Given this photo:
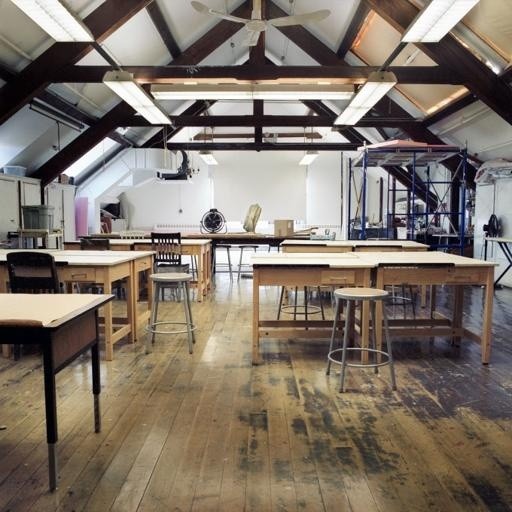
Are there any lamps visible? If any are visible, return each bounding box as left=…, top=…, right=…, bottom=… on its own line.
left=399, top=0, right=480, bottom=45
left=199, top=122, right=220, bottom=167
left=102, top=71, right=172, bottom=126
left=332, top=73, right=397, bottom=128
left=299, top=125, right=317, bottom=164
left=10, top=0, right=95, bottom=42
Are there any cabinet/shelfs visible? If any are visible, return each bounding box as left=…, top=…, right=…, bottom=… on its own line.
left=46, top=184, right=77, bottom=241
left=466, top=174, right=511, bottom=289
left=0, top=173, right=43, bottom=244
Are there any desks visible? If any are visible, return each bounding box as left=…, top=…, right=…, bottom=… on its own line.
left=0, top=290, right=120, bottom=493
left=238, top=203, right=511, bottom=366
left=1, top=248, right=157, bottom=359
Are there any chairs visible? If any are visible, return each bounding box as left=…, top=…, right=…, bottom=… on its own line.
left=5, top=251, right=65, bottom=364
left=62, top=226, right=214, bottom=303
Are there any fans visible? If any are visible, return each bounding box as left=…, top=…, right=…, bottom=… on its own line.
left=200, top=208, right=227, bottom=234
left=191, top=1, right=331, bottom=47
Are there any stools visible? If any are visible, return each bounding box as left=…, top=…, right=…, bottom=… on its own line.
left=214, top=244, right=234, bottom=283
left=148, top=272, right=198, bottom=355
left=325, top=287, right=402, bottom=393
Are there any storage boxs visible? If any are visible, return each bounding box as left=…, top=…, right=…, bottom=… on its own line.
left=3, top=165, right=27, bottom=177
left=22, top=204, right=55, bottom=234
